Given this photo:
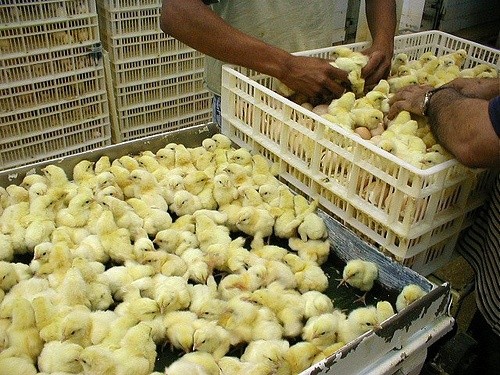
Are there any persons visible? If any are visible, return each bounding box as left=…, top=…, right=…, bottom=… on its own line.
left=386, top=75, right=500, bottom=374
left=156, top=1, right=399, bottom=134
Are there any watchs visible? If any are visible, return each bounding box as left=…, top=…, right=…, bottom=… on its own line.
left=419, top=86, right=449, bottom=118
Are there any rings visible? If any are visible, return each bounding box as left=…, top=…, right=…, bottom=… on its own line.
left=342, top=88, right=348, bottom=95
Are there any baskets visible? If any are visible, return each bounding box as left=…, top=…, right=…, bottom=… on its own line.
left=221, top=30, right=500, bottom=277
left=1, top=0, right=213, bottom=170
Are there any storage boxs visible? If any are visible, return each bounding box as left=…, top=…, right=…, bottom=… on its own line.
left=1, top=0, right=213, bottom=169
left=220, top=30, right=499, bottom=279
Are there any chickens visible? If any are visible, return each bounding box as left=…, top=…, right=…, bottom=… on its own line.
left=235, top=45, right=499, bottom=219
left=0, top=135, right=428, bottom=375
